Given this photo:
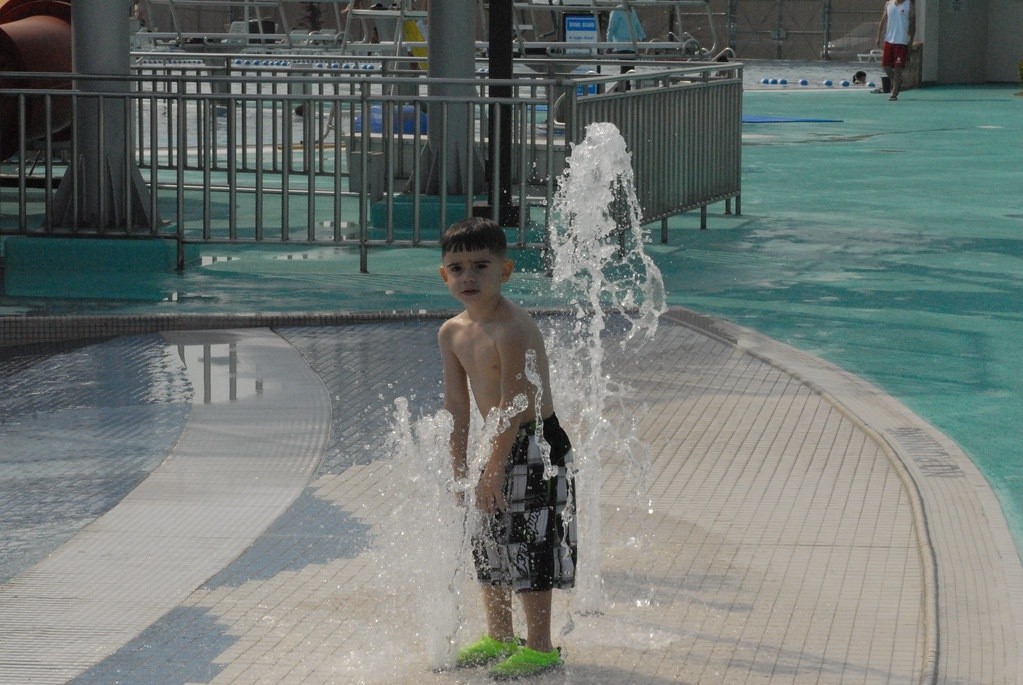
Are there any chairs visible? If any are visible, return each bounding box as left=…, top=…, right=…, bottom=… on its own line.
left=228, top=21, right=247, bottom=43
left=289, top=28, right=337, bottom=47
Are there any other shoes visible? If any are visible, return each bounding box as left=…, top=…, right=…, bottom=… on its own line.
left=888, top=96, right=897, bottom=101
left=486, top=646, right=564, bottom=681
left=456, top=633, right=527, bottom=669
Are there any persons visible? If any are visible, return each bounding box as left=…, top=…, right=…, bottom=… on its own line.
left=605, top=0, right=646, bottom=74
left=437, top=216, right=578, bottom=676
left=853, top=71, right=866, bottom=84
left=876, top=0, right=915, bottom=101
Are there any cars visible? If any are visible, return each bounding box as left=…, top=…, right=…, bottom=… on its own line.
left=176, top=37, right=220, bottom=49
left=820, top=20, right=881, bottom=60
